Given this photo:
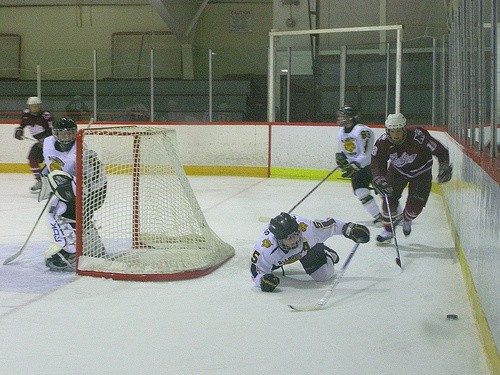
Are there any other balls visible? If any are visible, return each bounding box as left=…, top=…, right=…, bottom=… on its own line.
left=446, top=314, right=458, bottom=320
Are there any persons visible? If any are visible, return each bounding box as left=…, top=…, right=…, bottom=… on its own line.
left=250, top=213, right=370, bottom=292
left=370, top=114, right=453, bottom=242
left=15, top=97, right=106, bottom=271
left=335, top=105, right=404, bottom=228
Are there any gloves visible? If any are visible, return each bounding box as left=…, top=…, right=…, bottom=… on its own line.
left=437, top=161, right=452, bottom=183
left=54, top=180, right=75, bottom=202
left=371, top=176, right=393, bottom=197
left=15, top=126, right=23, bottom=140
left=260, top=273, right=280, bottom=292
left=32, top=132, right=44, bottom=139
left=335, top=151, right=360, bottom=178
left=342, top=221, right=370, bottom=244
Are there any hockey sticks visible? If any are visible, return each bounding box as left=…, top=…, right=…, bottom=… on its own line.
left=3, top=193, right=54, bottom=266
left=21, top=119, right=95, bottom=144
left=384, top=194, right=401, bottom=266
left=256, top=164, right=339, bottom=224
left=287, top=237, right=362, bottom=310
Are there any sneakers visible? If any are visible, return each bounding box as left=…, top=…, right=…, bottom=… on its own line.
left=323, top=245, right=339, bottom=264
left=45, top=248, right=82, bottom=272
left=29, top=176, right=42, bottom=194
left=376, top=219, right=396, bottom=243
left=402, top=216, right=412, bottom=237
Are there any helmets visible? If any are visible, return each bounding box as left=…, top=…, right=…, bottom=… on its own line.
left=268, top=212, right=301, bottom=254
left=26, top=97, right=42, bottom=105
left=52, top=116, right=77, bottom=151
left=385, top=113, right=407, bottom=144
left=335, top=104, right=353, bottom=127
